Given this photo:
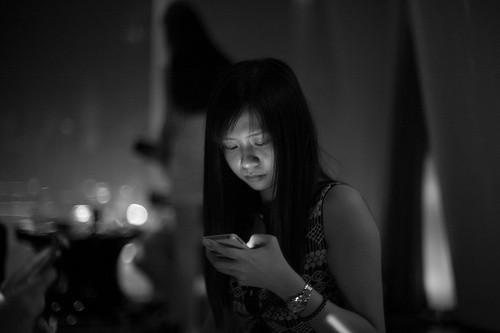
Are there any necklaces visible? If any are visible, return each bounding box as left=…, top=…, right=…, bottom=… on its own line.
left=255, top=207, right=264, bottom=219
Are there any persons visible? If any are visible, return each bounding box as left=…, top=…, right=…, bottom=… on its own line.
left=157, top=0, right=231, bottom=330
left=201, top=56, right=386, bottom=332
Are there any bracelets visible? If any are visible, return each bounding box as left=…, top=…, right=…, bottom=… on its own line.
left=300, top=294, right=328, bottom=322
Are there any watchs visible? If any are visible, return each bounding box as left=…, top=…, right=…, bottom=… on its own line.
left=286, top=283, right=312, bottom=314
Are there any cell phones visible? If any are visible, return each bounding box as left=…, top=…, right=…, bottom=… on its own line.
left=201, top=233, right=250, bottom=258
